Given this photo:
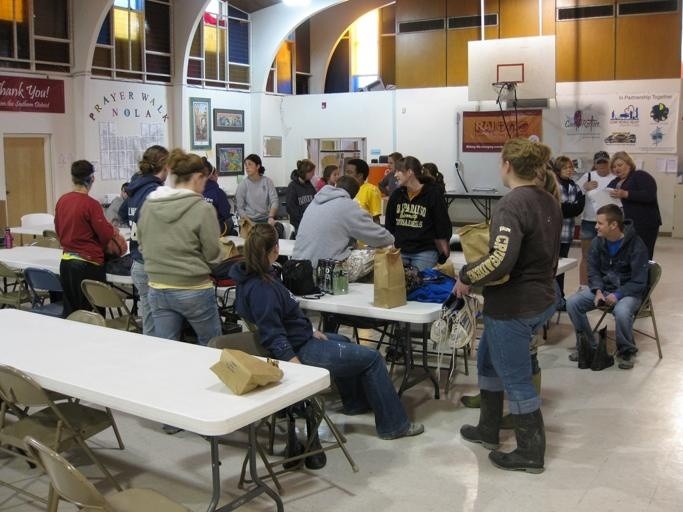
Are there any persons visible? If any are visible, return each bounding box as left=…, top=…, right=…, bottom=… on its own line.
left=104, top=143, right=453, bottom=364
left=229, top=221, right=426, bottom=441
left=137, top=149, right=226, bottom=436
left=575, top=150, right=624, bottom=293
left=451, top=137, right=563, bottom=474
left=550, top=157, right=584, bottom=313
left=563, top=202, right=648, bottom=370
left=455, top=142, right=560, bottom=429
left=606, top=150, right=662, bottom=262
left=51, top=158, right=119, bottom=321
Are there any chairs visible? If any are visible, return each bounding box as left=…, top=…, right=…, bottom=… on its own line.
left=593, top=260, right=663, bottom=360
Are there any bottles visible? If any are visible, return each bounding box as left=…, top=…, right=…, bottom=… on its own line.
left=316, top=258, right=350, bottom=297
left=4, top=228, right=12, bottom=249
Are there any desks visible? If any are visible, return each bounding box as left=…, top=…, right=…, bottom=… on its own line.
left=443, top=189, right=504, bottom=225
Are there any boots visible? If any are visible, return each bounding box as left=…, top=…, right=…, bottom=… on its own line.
left=589, top=323, right=615, bottom=371
left=460, top=393, right=482, bottom=409
left=576, top=324, right=595, bottom=370
left=502, top=368, right=541, bottom=430
left=303, top=400, right=328, bottom=471
left=280, top=402, right=306, bottom=472
left=489, top=408, right=546, bottom=475
left=461, top=388, right=504, bottom=450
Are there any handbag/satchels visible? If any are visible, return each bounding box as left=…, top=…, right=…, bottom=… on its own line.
left=281, top=258, right=322, bottom=296
left=104, top=231, right=129, bottom=258
left=401, top=263, right=425, bottom=297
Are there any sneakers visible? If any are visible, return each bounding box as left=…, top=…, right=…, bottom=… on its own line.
left=382, top=422, right=425, bottom=440
left=568, top=351, right=580, bottom=362
left=618, top=347, right=634, bottom=369
left=384, top=348, right=404, bottom=365
left=447, top=292, right=488, bottom=349
left=163, top=423, right=183, bottom=434
left=428, top=291, right=459, bottom=344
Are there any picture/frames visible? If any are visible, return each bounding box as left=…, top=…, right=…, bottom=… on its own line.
left=212, top=108, right=245, bottom=132
left=216, top=143, right=245, bottom=176
left=189, top=97, right=212, bottom=151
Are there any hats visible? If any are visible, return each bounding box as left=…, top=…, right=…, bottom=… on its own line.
left=594, top=151, right=610, bottom=162
